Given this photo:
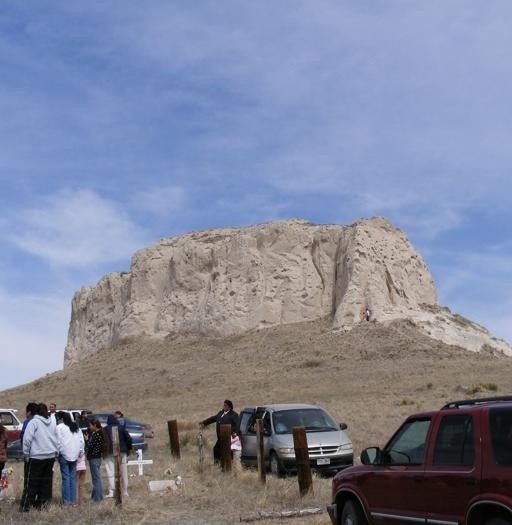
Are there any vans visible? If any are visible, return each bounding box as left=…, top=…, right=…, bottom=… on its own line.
left=234, top=402, right=357, bottom=479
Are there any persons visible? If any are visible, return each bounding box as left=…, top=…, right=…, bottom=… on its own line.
left=231, top=430, right=242, bottom=467
left=253, top=423, right=257, bottom=433
left=199, top=400, right=240, bottom=464
left=0, top=402, right=133, bottom=512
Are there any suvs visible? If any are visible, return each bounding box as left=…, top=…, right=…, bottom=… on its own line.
left=322, top=391, right=512, bottom=525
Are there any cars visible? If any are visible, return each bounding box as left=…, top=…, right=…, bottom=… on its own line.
left=0, top=408, right=155, bottom=461
